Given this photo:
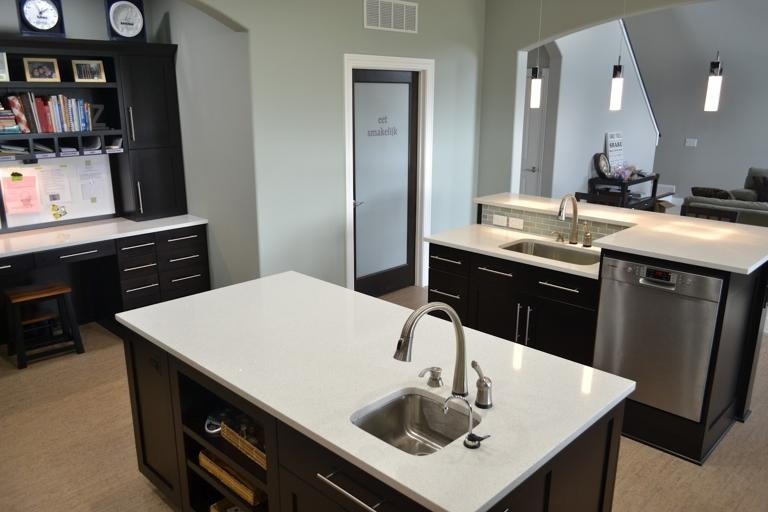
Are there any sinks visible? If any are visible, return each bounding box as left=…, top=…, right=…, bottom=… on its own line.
left=498, top=238, right=602, bottom=266
left=349, top=387, right=481, bottom=455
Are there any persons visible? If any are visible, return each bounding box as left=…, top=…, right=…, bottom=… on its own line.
left=31, top=65, right=56, bottom=78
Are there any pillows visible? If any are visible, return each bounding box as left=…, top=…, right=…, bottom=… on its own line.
left=691, top=187, right=735, bottom=200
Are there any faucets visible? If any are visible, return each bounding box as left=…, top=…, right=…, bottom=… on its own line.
left=555, top=194, right=579, bottom=244
left=393, top=300, right=469, bottom=398
left=442, top=396, right=492, bottom=449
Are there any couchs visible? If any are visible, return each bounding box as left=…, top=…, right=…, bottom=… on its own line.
left=679, top=162, right=767, bottom=231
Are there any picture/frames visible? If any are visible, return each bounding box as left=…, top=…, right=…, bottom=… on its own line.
left=73, top=59, right=109, bottom=85
left=22, top=55, right=61, bottom=85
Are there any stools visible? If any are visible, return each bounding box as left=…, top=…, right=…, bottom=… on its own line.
left=0, top=281, right=85, bottom=368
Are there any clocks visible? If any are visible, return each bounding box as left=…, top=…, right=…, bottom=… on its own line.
left=15, top=0, right=67, bottom=39
left=594, top=153, right=611, bottom=176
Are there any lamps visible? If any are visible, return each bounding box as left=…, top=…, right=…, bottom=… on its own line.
left=701, top=0, right=727, bottom=114
left=606, top=0, right=627, bottom=112
left=528, top=0, right=544, bottom=110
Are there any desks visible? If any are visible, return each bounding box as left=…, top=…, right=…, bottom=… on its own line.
left=0, top=220, right=211, bottom=362
left=588, top=171, right=660, bottom=211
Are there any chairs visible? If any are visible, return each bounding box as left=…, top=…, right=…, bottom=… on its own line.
left=680, top=202, right=740, bottom=223
left=575, top=191, right=621, bottom=208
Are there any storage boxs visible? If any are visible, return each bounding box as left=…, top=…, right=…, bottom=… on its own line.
left=631, top=193, right=675, bottom=215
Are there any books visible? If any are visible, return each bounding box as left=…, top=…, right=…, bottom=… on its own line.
left=0, top=89, right=123, bottom=157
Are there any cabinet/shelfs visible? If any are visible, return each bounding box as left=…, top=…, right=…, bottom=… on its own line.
left=166, top=355, right=277, bottom=512
left=463, top=251, right=603, bottom=367
left=122, top=329, right=181, bottom=512
left=120, top=43, right=188, bottom=224
left=0, top=40, right=125, bottom=169
left=427, top=244, right=468, bottom=326
left=277, top=422, right=435, bottom=512
